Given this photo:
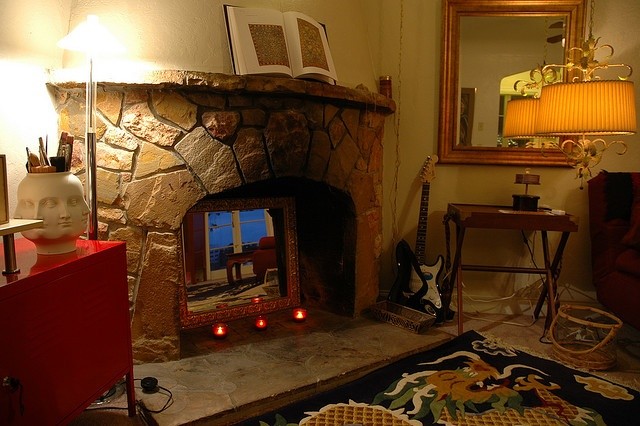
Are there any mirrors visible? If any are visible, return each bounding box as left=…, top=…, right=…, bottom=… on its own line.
left=437, top=1, right=591, bottom=168
left=179, top=196, right=301, bottom=330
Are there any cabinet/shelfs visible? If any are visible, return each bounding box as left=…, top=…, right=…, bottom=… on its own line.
left=0, top=233, right=136, bottom=426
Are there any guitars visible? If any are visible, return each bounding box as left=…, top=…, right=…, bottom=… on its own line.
left=388, top=154, right=445, bottom=316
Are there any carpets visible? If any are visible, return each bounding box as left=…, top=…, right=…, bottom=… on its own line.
left=183, top=329, right=640, bottom=425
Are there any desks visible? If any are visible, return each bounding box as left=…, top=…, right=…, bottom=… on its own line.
left=445, top=198, right=579, bottom=336
left=226, top=250, right=254, bottom=286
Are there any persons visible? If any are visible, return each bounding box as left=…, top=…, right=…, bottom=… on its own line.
left=13, top=173, right=91, bottom=255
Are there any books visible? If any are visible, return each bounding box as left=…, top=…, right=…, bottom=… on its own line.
left=223, top=5, right=338, bottom=84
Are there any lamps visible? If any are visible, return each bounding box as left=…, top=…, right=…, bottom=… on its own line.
left=56, top=14, right=125, bottom=242
left=503, top=16, right=556, bottom=142
left=533, top=1, right=638, bottom=191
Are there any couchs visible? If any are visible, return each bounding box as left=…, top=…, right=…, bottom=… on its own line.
left=253, top=235, right=278, bottom=275
left=588, top=171, right=640, bottom=329
